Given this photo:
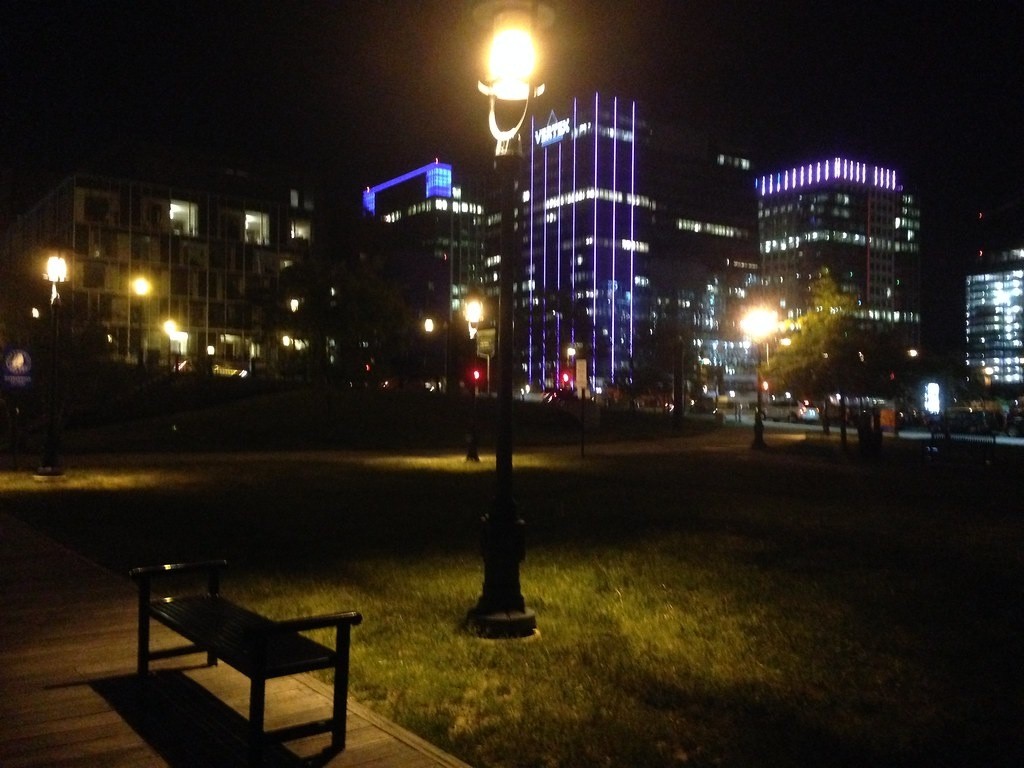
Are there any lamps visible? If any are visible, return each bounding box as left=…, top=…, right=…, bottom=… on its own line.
left=46, top=256, right=66, bottom=282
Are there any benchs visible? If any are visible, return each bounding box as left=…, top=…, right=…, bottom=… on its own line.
left=130, top=559, right=363, bottom=768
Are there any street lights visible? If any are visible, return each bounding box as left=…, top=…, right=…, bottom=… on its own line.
left=37, top=256, right=72, bottom=478
left=131, top=277, right=150, bottom=363
left=473, top=19, right=543, bottom=638
left=743, top=305, right=779, bottom=450
